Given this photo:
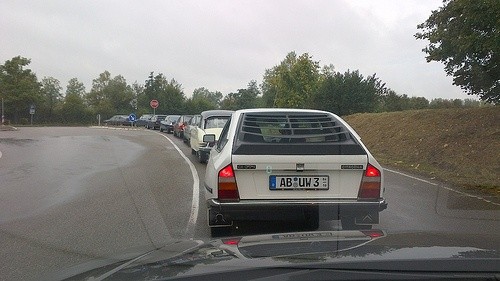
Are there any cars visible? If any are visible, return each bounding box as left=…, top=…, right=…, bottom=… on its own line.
left=204, top=108, right=387, bottom=238
left=182, top=114, right=202, bottom=145
left=172, top=114, right=194, bottom=139
left=160, top=115, right=181, bottom=134
left=144, top=115, right=166, bottom=130
left=190, top=109, right=236, bottom=163
left=107, top=115, right=132, bottom=126
left=134, top=115, right=155, bottom=128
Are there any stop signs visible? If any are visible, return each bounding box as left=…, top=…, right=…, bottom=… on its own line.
left=150, top=100, right=159, bottom=108
